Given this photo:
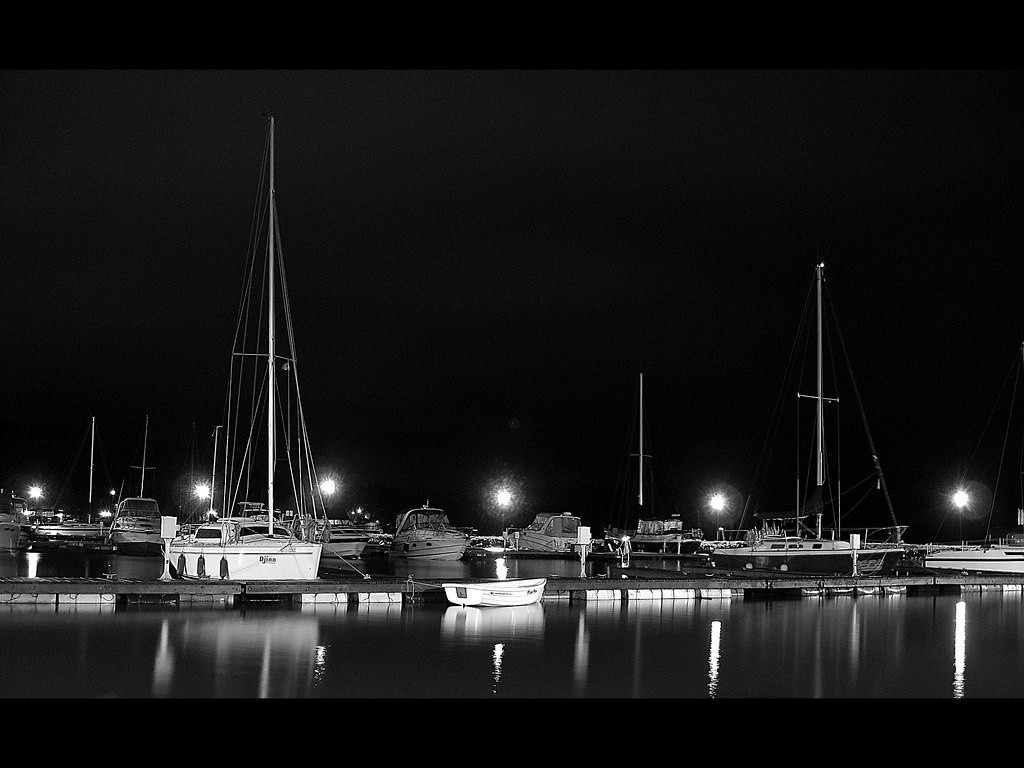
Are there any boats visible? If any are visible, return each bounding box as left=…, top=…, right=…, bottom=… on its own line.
left=443, top=603, right=545, bottom=633
left=440, top=576, right=547, bottom=607
left=0, top=115, right=1024, bottom=586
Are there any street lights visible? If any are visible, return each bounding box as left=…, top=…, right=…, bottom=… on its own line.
left=492, top=485, right=512, bottom=531
left=706, top=491, right=728, bottom=530
left=951, top=488, right=970, bottom=544
